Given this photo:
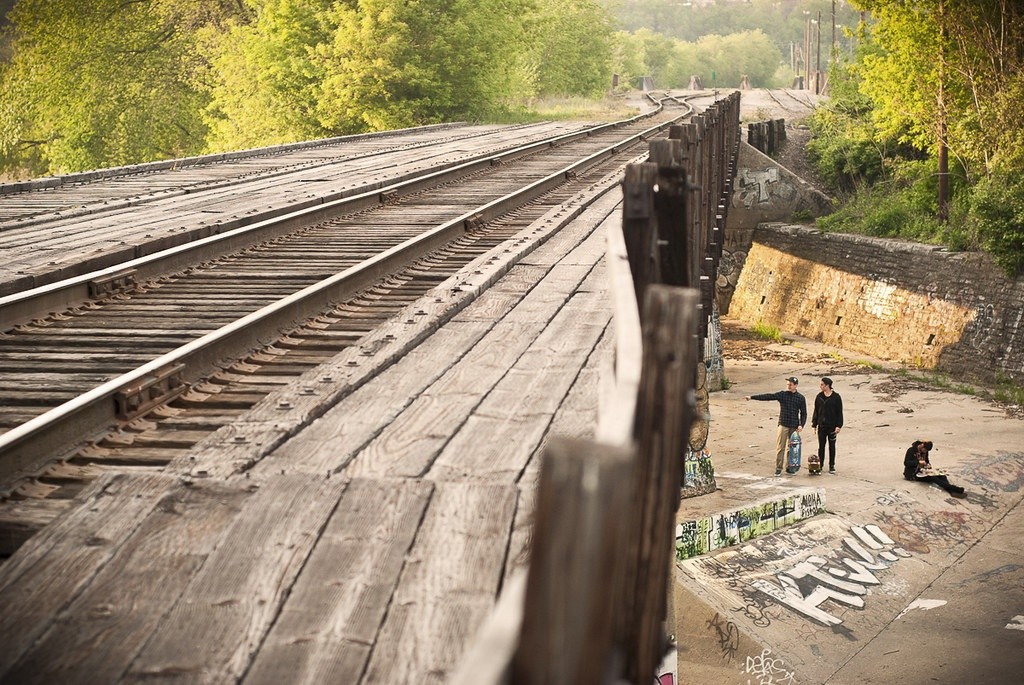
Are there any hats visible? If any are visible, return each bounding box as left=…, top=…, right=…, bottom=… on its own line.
left=785, top=377, right=798, bottom=384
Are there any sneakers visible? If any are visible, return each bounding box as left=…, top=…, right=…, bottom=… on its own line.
left=774, top=469, right=782, bottom=476
left=829, top=468, right=837, bottom=474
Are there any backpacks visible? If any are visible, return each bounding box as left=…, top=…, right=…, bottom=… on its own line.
left=809, top=455, right=820, bottom=473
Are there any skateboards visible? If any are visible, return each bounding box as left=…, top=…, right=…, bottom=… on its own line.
left=786, top=431, right=802, bottom=474
left=916, top=468, right=950, bottom=478
left=808, top=454, right=822, bottom=476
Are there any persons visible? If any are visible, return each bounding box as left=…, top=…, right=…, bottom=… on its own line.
left=904, top=441, right=967, bottom=499
left=742, top=377, right=807, bottom=476
left=812, top=378, right=843, bottom=471
